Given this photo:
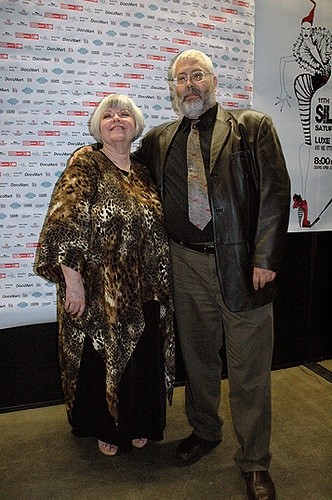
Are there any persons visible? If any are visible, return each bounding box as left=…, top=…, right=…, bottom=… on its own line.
left=36, top=95, right=177, bottom=455
left=69, top=49, right=292, bottom=500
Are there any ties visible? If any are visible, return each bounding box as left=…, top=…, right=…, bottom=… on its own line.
left=186, top=118, right=212, bottom=231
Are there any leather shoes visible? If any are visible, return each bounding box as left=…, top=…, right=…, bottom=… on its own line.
left=174, top=431, right=219, bottom=464
left=242, top=470, right=277, bottom=500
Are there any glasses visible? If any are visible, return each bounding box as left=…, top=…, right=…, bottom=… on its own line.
left=171, top=70, right=212, bottom=86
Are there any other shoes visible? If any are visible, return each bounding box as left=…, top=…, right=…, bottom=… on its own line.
left=132, top=438, right=148, bottom=448
left=93, top=440, right=118, bottom=455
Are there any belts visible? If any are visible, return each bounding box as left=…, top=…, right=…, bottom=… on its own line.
left=172, top=238, right=216, bottom=253
